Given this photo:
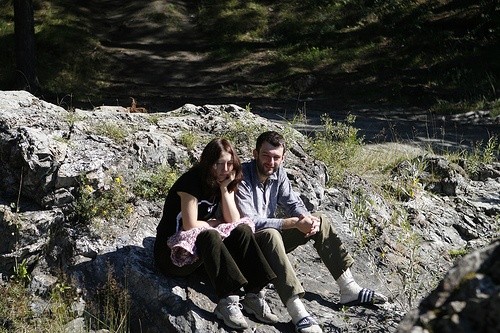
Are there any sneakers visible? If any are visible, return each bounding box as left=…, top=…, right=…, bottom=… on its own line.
left=214, top=295, right=249, bottom=329
left=240, top=291, right=279, bottom=325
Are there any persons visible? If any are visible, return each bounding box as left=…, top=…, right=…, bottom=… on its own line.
left=234, top=131, right=388, bottom=333
left=153, top=136, right=279, bottom=330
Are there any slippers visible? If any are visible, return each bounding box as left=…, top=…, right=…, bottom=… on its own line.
left=290, top=316, right=320, bottom=333
left=343, top=288, right=385, bottom=306
